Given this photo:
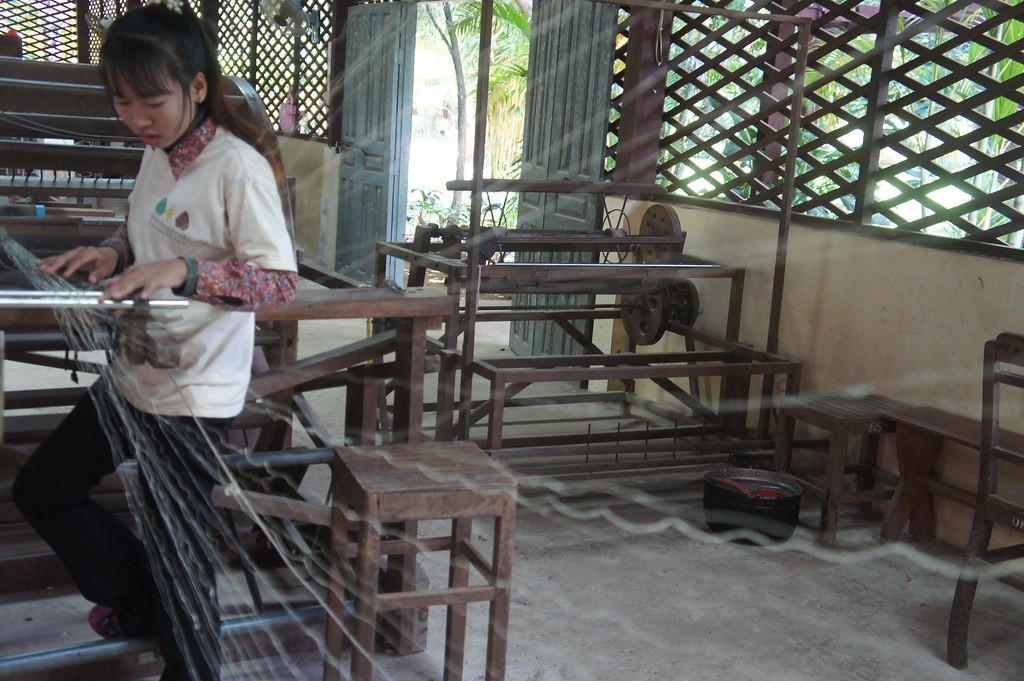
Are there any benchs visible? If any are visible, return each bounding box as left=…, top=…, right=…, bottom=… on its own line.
left=880, top=407, right=1024, bottom=544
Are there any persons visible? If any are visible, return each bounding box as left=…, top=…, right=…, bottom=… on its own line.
left=10, top=4, right=298, bottom=680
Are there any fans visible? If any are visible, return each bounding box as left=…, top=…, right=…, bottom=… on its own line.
left=260, top=0, right=320, bottom=44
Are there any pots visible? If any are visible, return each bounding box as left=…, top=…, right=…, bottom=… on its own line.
left=702, top=450, right=803, bottom=546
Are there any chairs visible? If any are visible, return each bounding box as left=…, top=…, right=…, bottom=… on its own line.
left=946, top=331, right=1024, bottom=669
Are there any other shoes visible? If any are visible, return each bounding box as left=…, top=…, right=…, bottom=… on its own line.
left=89, top=604, right=126, bottom=639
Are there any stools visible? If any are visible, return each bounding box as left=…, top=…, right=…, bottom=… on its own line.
left=775, top=392, right=912, bottom=545
left=321, top=440, right=517, bottom=681
